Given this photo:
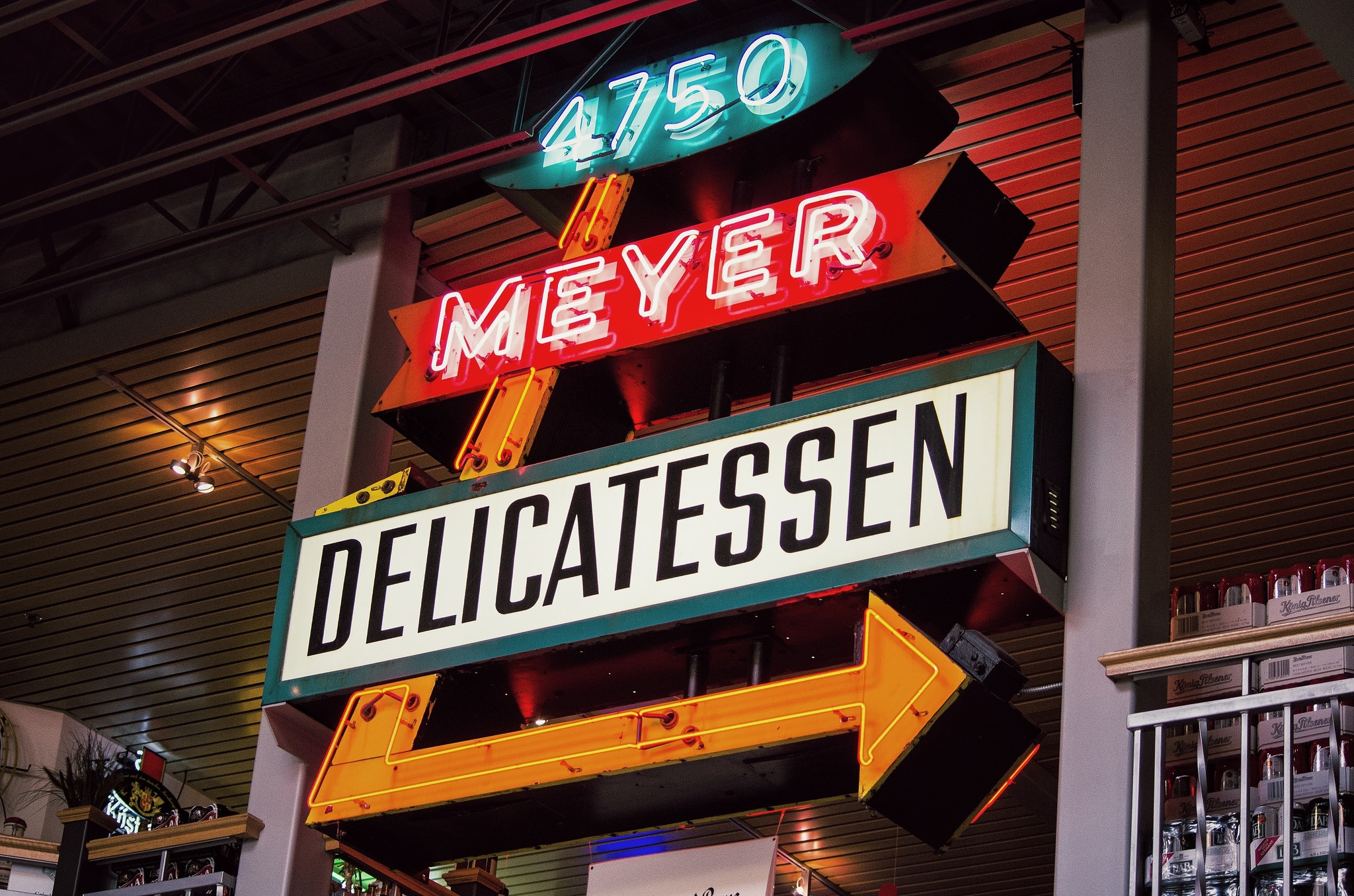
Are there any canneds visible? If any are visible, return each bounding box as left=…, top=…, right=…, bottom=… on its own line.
left=1161, top=702, right=1354, bottom=896
left=1171, top=555, right=1354, bottom=618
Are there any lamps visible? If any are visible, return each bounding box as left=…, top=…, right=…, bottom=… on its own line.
left=170, top=458, right=191, bottom=477
left=193, top=476, right=215, bottom=494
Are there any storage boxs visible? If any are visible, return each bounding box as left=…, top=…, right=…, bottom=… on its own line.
left=1144, top=581, right=1354, bottom=887
